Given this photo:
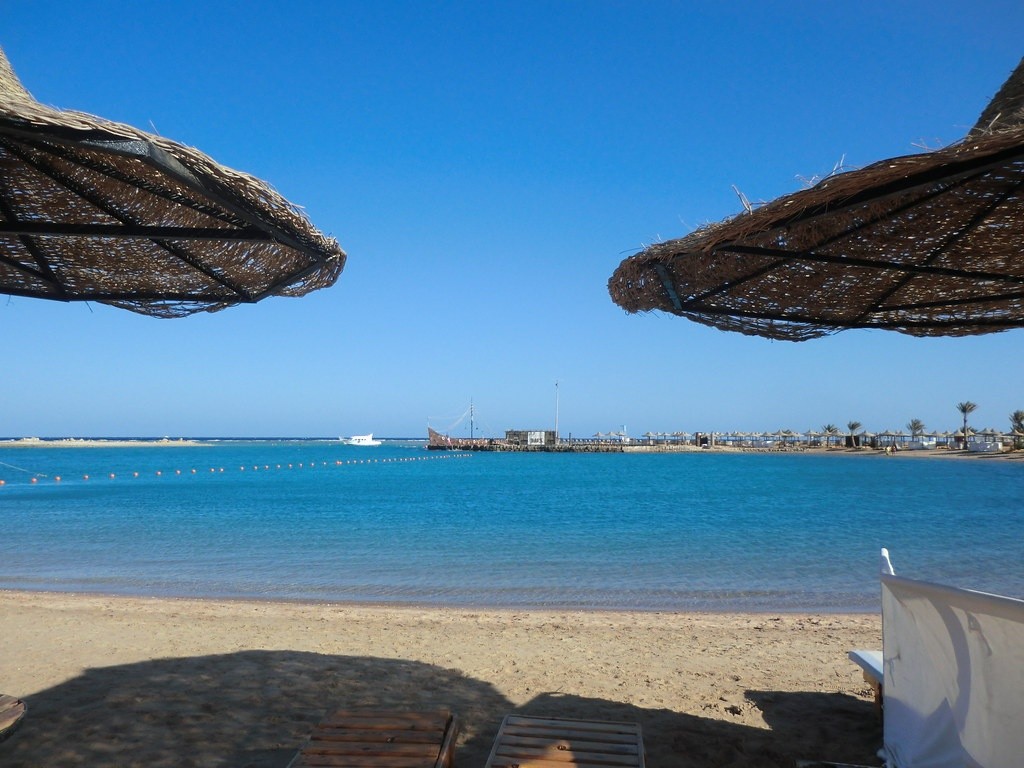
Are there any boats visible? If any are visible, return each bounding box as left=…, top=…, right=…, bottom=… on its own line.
left=428, top=397, right=506, bottom=453
left=340, top=432, right=382, bottom=446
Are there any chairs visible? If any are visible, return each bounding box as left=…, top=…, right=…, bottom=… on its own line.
left=484, top=714, right=647, bottom=768
left=286, top=709, right=461, bottom=768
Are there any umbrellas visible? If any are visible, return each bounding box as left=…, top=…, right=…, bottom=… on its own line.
left=605, top=59, right=1024, bottom=341
left=0, top=43, right=346, bottom=321
left=591, top=427, right=1006, bottom=447
